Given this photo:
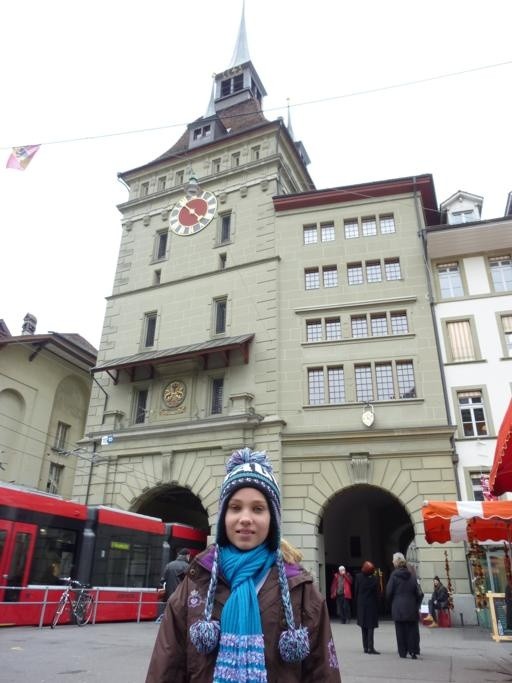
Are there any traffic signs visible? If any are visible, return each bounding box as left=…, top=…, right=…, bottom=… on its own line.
left=101, top=434, right=113, bottom=445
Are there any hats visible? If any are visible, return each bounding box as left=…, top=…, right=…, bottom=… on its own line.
left=434, top=576, right=440, bottom=582
left=189, top=447, right=311, bottom=664
left=179, top=548, right=190, bottom=555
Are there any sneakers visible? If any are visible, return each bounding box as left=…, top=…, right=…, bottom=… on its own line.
left=424, top=613, right=433, bottom=621
left=411, top=652, right=417, bottom=659
left=364, top=648, right=368, bottom=652
left=368, top=649, right=379, bottom=654
left=429, top=621, right=438, bottom=627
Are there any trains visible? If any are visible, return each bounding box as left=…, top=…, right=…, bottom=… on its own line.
left=0, top=482, right=207, bottom=626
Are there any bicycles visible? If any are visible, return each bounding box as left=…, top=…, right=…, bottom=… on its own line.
left=50, top=576, right=95, bottom=628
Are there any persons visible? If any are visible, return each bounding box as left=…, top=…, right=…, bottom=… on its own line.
left=384, top=551, right=421, bottom=659
left=145, top=448, right=342, bottom=683
left=407, top=562, right=424, bottom=653
left=330, top=564, right=353, bottom=624
left=160, top=547, right=192, bottom=601
left=353, top=560, right=381, bottom=655
left=424, top=575, right=449, bottom=627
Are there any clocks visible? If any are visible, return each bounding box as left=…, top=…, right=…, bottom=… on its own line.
left=167, top=191, right=217, bottom=234
